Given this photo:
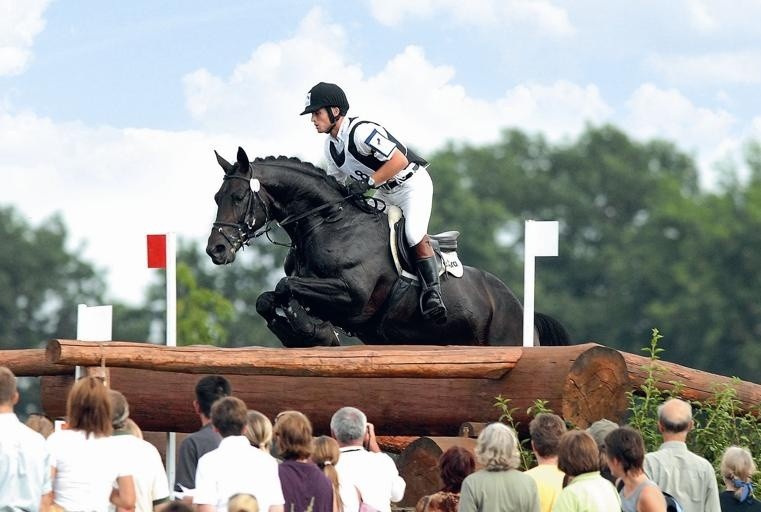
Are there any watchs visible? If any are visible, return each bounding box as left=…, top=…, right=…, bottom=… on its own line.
left=368, top=176, right=375, bottom=188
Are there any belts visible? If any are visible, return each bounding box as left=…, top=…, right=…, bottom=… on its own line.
left=376, top=163, right=419, bottom=192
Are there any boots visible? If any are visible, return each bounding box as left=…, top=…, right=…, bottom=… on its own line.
left=413, top=255, right=448, bottom=325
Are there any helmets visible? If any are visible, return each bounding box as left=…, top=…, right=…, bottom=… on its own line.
left=299, top=82, right=349, bottom=115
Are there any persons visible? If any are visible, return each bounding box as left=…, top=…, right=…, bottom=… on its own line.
left=26, top=376, right=170, bottom=512
left=175, top=396, right=361, bottom=512
left=643, top=397, right=721, bottom=512
left=719, top=447, right=760, bottom=512
left=331, top=406, right=406, bottom=512
left=416, top=412, right=667, bottom=512
left=1, top=367, right=48, bottom=512
left=173, top=376, right=230, bottom=494
left=300, top=82, right=448, bottom=326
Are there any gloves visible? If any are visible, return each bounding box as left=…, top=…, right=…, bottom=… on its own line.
left=349, top=177, right=376, bottom=196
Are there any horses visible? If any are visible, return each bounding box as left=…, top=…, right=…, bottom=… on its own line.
left=205, top=146, right=568, bottom=349
